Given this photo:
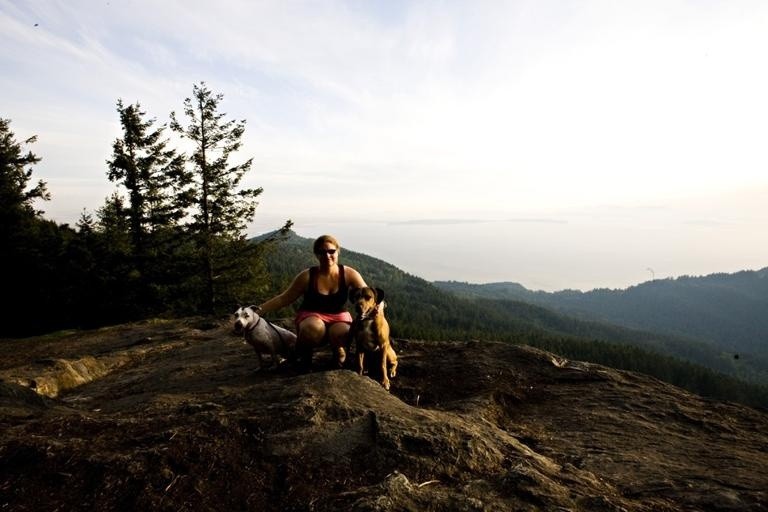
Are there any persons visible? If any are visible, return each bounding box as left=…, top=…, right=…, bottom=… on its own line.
left=255, top=234, right=368, bottom=370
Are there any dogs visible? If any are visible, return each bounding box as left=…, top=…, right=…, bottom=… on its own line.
left=348, top=285, right=398, bottom=391
left=233, top=302, right=298, bottom=377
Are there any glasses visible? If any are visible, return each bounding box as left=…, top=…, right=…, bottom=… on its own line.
left=317, top=249, right=336, bottom=255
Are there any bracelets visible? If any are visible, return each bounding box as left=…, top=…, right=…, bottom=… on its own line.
left=259, top=304, right=264, bottom=316
